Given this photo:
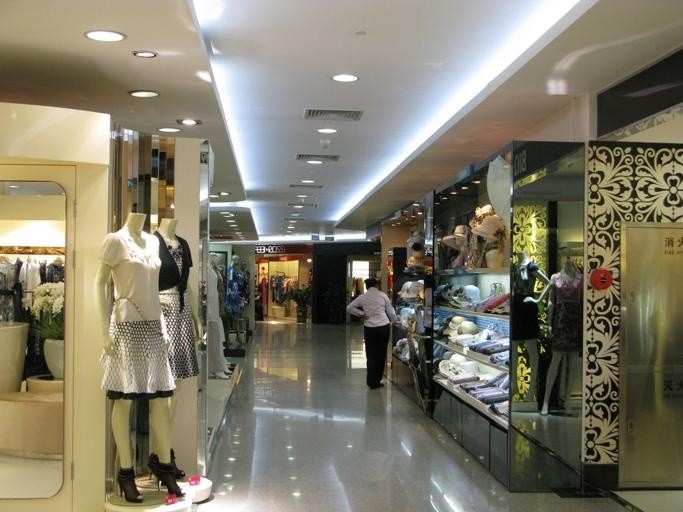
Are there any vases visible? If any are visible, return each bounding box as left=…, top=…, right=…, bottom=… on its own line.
left=40, top=337, right=65, bottom=383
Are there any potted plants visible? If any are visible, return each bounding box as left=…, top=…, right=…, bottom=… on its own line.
left=288, top=283, right=311, bottom=324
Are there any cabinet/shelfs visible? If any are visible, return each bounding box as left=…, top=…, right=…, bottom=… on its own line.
left=385, top=245, right=425, bottom=411
left=432, top=259, right=513, bottom=492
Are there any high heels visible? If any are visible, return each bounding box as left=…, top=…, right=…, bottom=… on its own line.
left=117, top=469, right=143, bottom=503
left=147, top=449, right=186, bottom=496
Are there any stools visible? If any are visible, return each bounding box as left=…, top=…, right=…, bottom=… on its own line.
left=0, top=391, right=66, bottom=462
left=272, top=306, right=285, bottom=318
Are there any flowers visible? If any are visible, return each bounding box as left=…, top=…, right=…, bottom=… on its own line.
left=27, top=280, right=67, bottom=338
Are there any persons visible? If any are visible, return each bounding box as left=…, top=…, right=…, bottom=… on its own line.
left=225, top=255, right=243, bottom=344
left=94, top=214, right=184, bottom=503
left=510, top=252, right=553, bottom=402
left=346, top=278, right=399, bottom=390
left=541, top=262, right=583, bottom=416
left=237, top=258, right=251, bottom=319
left=207, top=264, right=232, bottom=379
left=150, top=219, right=204, bottom=480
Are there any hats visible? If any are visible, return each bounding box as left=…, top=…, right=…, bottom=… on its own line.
left=441, top=283, right=480, bottom=308
left=441, top=225, right=470, bottom=252
left=443, top=317, right=479, bottom=336
left=437, top=354, right=484, bottom=378
left=471, top=216, right=504, bottom=241
left=397, top=279, right=425, bottom=300
